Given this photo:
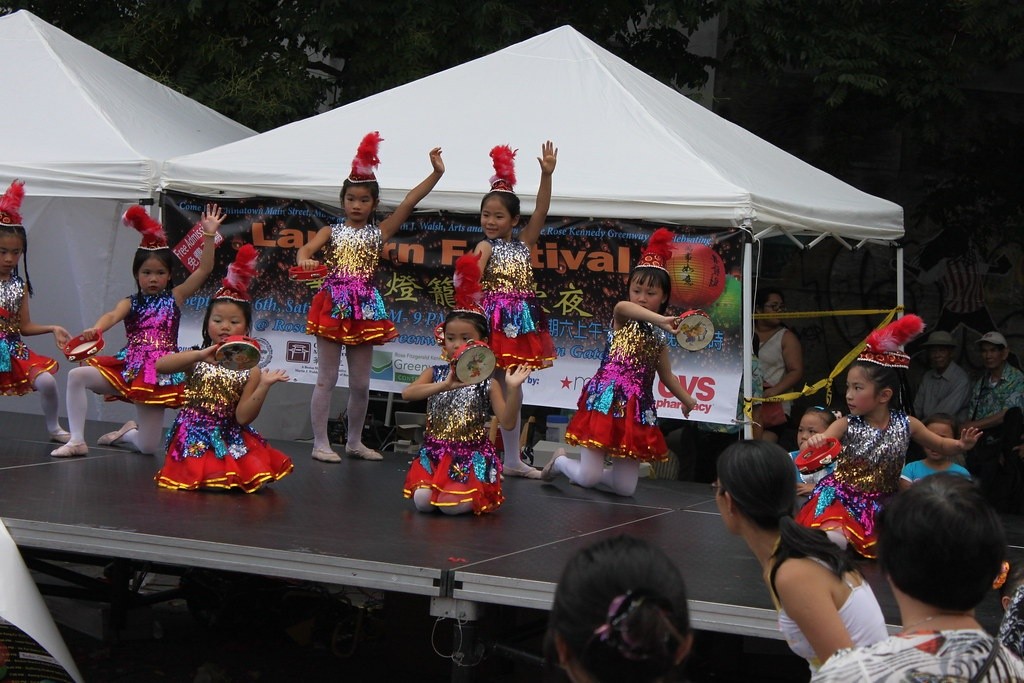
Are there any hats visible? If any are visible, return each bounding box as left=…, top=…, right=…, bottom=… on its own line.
left=975, top=331, right=1008, bottom=349
left=919, top=331, right=957, bottom=350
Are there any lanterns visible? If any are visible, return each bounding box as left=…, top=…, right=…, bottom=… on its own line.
left=665, top=241, right=741, bottom=334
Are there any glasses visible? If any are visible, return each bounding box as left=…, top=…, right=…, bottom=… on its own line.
left=711, top=482, right=740, bottom=504
left=764, top=303, right=786, bottom=312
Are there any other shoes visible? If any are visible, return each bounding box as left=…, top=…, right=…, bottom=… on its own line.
left=499, top=473, right=505, bottom=482
left=97, top=421, right=138, bottom=446
left=346, top=444, right=383, bottom=461
left=503, top=465, right=542, bottom=479
left=311, top=448, right=341, bottom=463
left=49, top=432, right=71, bottom=444
left=541, top=448, right=567, bottom=482
left=51, top=442, right=88, bottom=457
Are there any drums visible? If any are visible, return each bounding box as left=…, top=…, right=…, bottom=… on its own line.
left=214, top=334, right=262, bottom=371
left=671, top=309, right=715, bottom=352
left=287, top=265, right=329, bottom=282
left=446, top=339, right=496, bottom=385
left=63, top=329, right=106, bottom=364
left=794, top=437, right=845, bottom=476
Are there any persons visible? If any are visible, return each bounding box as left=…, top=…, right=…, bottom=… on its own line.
left=911, top=329, right=1024, bottom=515
left=787, top=404, right=847, bottom=517
left=152, top=244, right=295, bottom=494
left=473, top=137, right=559, bottom=480
left=541, top=535, right=693, bottom=683
left=295, top=130, right=445, bottom=460
left=898, top=413, right=973, bottom=493
left=710, top=440, right=889, bottom=683
left=544, top=228, right=696, bottom=497
left=745, top=287, right=804, bottom=446
left=402, top=248, right=532, bottom=516
left=49, top=202, right=226, bottom=457
left=810, top=470, right=1024, bottom=683
left=0, top=178, right=72, bottom=442
left=787, top=313, right=985, bottom=566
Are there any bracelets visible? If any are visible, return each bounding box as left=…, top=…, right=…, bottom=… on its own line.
left=202, top=231, right=218, bottom=238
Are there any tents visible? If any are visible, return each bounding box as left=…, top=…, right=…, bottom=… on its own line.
left=0, top=11, right=261, bottom=415
left=149, top=24, right=905, bottom=445
